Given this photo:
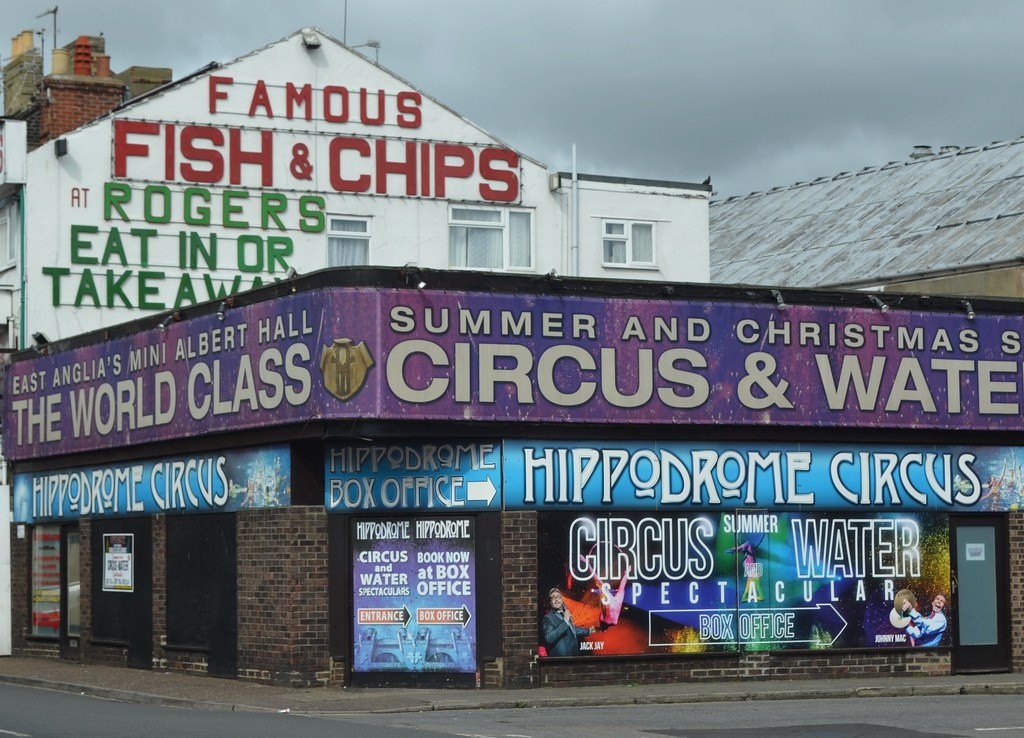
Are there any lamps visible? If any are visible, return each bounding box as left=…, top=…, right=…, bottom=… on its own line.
left=870, top=295, right=889, bottom=313
left=158, top=312, right=181, bottom=327
left=402, top=270, right=426, bottom=288
left=770, top=290, right=786, bottom=310
left=216, top=298, right=234, bottom=320
left=962, top=300, right=977, bottom=319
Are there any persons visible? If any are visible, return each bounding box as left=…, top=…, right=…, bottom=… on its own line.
left=542, top=587, right=596, bottom=657
left=900, top=594, right=947, bottom=648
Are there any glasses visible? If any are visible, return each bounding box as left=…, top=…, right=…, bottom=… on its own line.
left=550, top=594, right=561, bottom=601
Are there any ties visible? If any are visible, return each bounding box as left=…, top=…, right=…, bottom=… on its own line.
left=929, top=612, right=935, bottom=620
left=561, top=612, right=574, bottom=631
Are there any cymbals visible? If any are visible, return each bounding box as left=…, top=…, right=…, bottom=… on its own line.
left=889, top=607, right=912, bottom=629
left=894, top=589, right=917, bottom=618
